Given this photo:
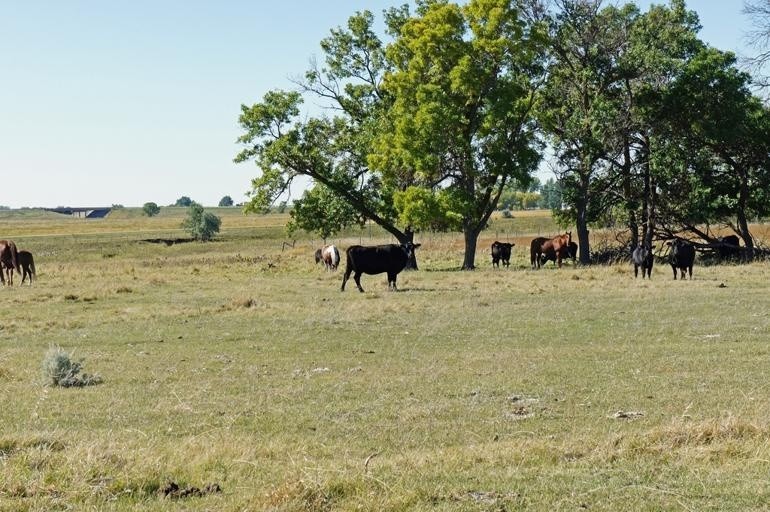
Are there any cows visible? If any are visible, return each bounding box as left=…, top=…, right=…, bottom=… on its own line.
left=490, top=239, right=514, bottom=268
left=666, top=237, right=695, bottom=281
left=540, top=242, right=579, bottom=267
left=339, top=241, right=422, bottom=295
left=716, top=235, right=740, bottom=260
left=632, top=242, right=656, bottom=280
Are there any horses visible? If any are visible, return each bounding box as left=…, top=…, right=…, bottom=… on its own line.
left=530, top=230, right=572, bottom=271
left=312, top=243, right=341, bottom=273
left=0, top=239, right=37, bottom=287
